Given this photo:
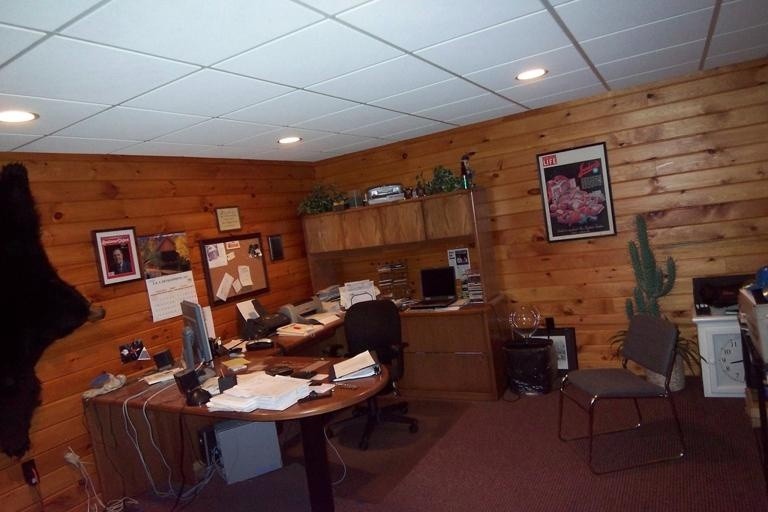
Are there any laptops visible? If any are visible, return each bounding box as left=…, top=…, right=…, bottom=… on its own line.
left=409, top=266, right=457, bottom=308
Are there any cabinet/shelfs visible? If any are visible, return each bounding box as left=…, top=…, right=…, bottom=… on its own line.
left=301, top=186, right=489, bottom=256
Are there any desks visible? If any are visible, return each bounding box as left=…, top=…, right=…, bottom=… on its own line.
left=322, top=295, right=514, bottom=401
left=80, top=314, right=388, bottom=512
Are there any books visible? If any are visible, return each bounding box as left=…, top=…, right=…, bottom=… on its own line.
left=276, top=323, right=323, bottom=336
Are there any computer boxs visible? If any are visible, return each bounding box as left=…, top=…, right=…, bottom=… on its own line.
left=212, top=420, right=283, bottom=485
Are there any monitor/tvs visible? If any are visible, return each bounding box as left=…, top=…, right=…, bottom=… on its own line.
left=179, top=300, right=216, bottom=382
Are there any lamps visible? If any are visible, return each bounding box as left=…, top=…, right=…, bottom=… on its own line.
left=277, top=136, right=303, bottom=145
left=515, top=68, right=549, bottom=82
left=0, top=110, right=40, bottom=123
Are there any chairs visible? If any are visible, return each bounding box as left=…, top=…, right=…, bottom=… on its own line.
left=324, top=300, right=420, bottom=450
left=557, top=311, right=686, bottom=477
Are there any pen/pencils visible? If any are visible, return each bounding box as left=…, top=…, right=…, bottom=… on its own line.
left=220, top=369, right=224, bottom=378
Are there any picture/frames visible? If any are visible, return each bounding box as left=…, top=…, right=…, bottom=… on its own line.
left=264, top=233, right=286, bottom=263
left=535, top=141, right=618, bottom=244
left=91, top=225, right=145, bottom=287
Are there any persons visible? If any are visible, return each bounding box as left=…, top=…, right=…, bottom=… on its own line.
left=107, top=247, right=131, bottom=274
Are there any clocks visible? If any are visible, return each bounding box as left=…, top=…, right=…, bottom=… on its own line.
left=720, top=338, right=746, bottom=384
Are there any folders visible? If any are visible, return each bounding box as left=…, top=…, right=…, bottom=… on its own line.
left=329, top=349, right=382, bottom=381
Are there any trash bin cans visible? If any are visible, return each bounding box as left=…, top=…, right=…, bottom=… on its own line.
left=502, top=338, right=552, bottom=396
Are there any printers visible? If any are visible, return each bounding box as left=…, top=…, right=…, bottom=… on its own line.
left=238, top=299, right=290, bottom=340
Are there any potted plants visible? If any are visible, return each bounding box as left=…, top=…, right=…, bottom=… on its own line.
left=608, top=211, right=708, bottom=393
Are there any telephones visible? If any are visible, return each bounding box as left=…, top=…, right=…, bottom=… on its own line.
left=246, top=338, right=273, bottom=350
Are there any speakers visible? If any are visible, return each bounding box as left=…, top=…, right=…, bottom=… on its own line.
left=152, top=349, right=175, bottom=369
left=173, top=369, right=199, bottom=395
left=197, top=428, right=216, bottom=465
